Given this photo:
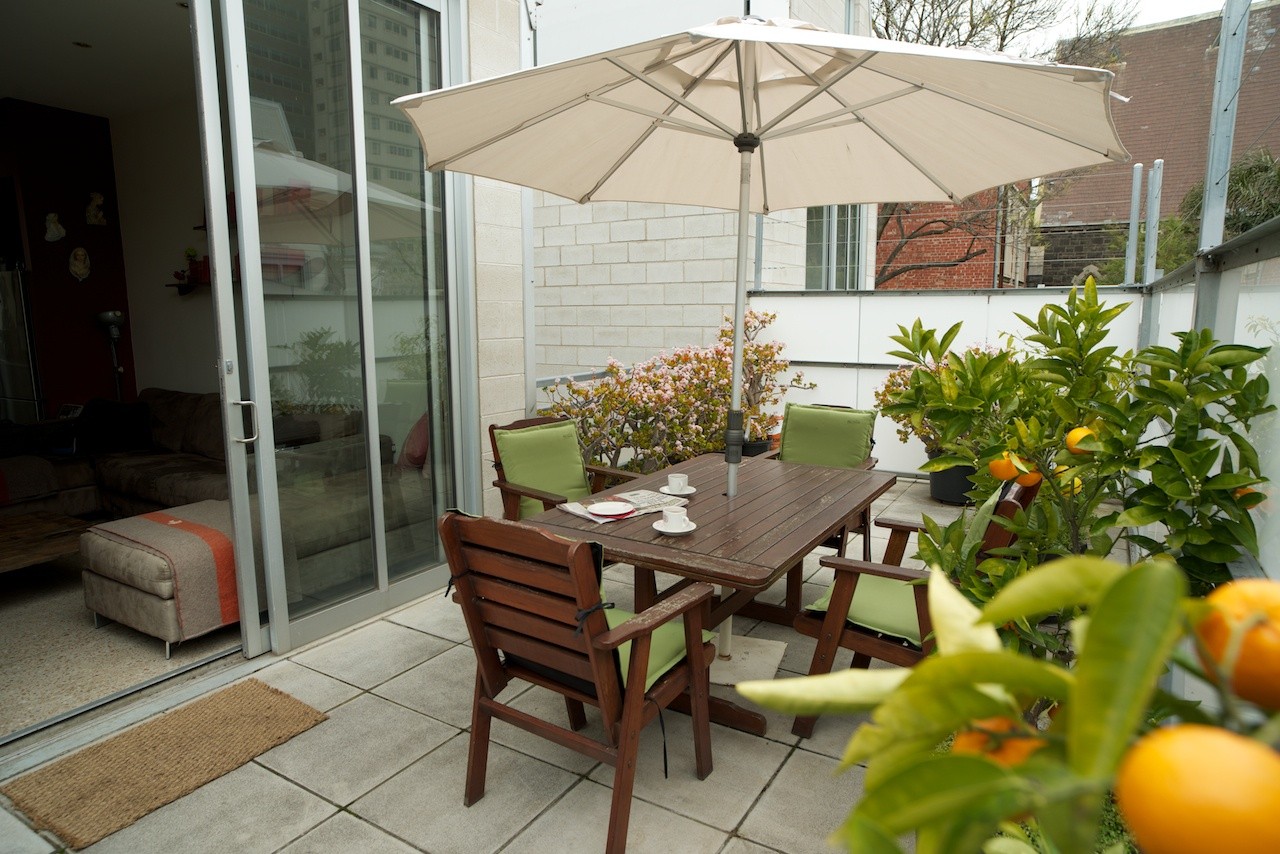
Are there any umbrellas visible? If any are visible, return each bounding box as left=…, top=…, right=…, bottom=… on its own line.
left=393, top=1, right=1135, bottom=660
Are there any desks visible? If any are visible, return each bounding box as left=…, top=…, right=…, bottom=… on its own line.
left=520, top=452, right=898, bottom=735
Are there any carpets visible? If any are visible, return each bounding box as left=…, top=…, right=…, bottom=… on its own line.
left=0, top=676, right=328, bottom=851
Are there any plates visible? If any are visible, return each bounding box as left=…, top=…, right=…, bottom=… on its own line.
left=652, top=519, right=697, bottom=536
left=587, top=502, right=634, bottom=515
left=660, top=486, right=696, bottom=496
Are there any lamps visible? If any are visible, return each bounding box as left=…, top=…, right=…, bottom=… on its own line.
left=99, top=311, right=125, bottom=400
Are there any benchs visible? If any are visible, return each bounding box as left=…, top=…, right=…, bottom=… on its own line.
left=80, top=464, right=438, bottom=660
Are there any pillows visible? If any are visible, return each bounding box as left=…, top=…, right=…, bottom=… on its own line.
left=401, top=411, right=429, bottom=468
left=106, top=399, right=154, bottom=452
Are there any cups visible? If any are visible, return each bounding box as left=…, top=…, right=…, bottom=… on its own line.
left=668, top=473, right=688, bottom=493
left=662, top=506, right=688, bottom=530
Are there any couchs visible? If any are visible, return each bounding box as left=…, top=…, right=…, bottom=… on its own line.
left=0, top=388, right=392, bottom=523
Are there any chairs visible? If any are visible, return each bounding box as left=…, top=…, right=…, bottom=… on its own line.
left=438, top=401, right=1043, bottom=854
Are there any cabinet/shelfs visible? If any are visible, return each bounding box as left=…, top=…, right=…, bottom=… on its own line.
left=164, top=223, right=242, bottom=295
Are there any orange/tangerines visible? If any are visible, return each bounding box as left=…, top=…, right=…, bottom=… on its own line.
left=1236, top=487, right=1257, bottom=510
left=952, top=576, right=1280, bottom=854
left=989, top=428, right=1098, bottom=494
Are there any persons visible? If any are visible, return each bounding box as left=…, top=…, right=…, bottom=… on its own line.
left=71, top=248, right=88, bottom=274
left=45, top=213, right=65, bottom=241
left=184, top=248, right=201, bottom=284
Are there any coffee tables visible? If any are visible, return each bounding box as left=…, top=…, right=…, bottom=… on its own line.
left=0, top=509, right=98, bottom=574
